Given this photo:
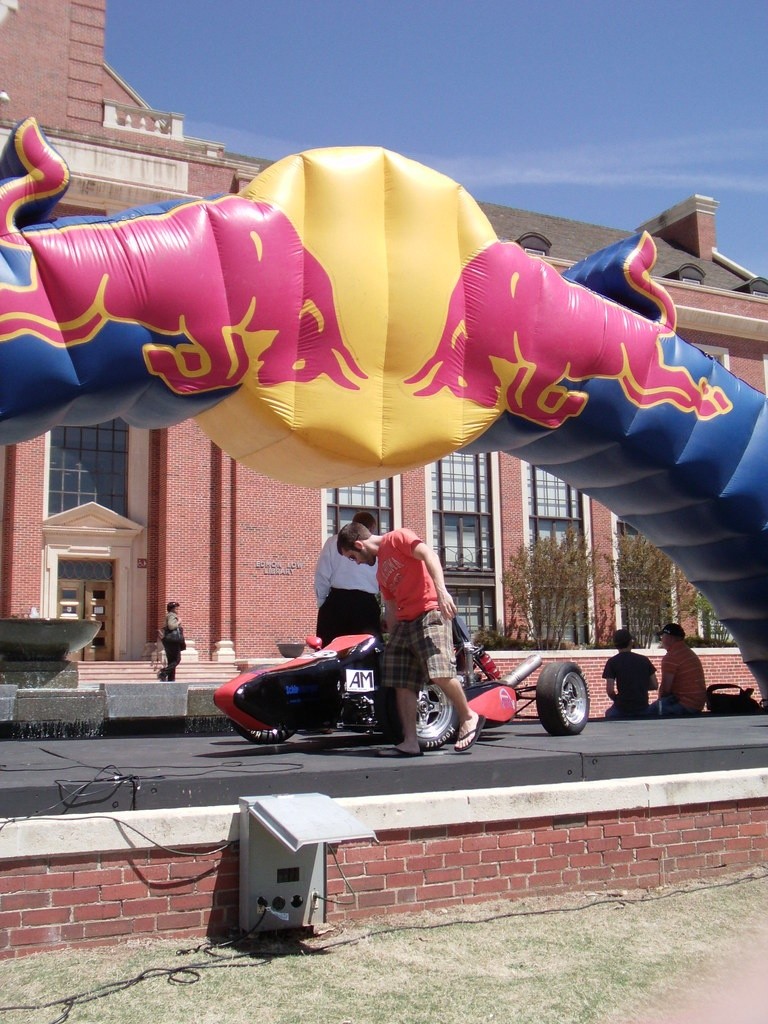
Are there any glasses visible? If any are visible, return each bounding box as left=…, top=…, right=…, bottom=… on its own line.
left=348, top=534, right=361, bottom=561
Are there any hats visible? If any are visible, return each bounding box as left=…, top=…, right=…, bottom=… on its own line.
left=655, top=623, right=685, bottom=641
left=613, top=629, right=636, bottom=645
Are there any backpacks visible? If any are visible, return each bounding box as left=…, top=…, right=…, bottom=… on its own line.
left=705, top=684, right=765, bottom=715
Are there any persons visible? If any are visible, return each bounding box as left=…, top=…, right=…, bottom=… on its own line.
left=336, top=522, right=485, bottom=759
left=156, top=601, right=182, bottom=682
left=648, top=623, right=707, bottom=716
left=602, top=629, right=658, bottom=718
left=315, top=511, right=383, bottom=651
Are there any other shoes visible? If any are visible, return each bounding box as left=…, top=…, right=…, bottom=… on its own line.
left=157, top=671, right=166, bottom=681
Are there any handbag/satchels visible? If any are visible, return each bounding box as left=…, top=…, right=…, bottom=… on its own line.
left=161, top=614, right=186, bottom=653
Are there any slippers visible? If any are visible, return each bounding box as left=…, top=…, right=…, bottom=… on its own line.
left=375, top=746, right=424, bottom=758
left=454, top=715, right=485, bottom=752
